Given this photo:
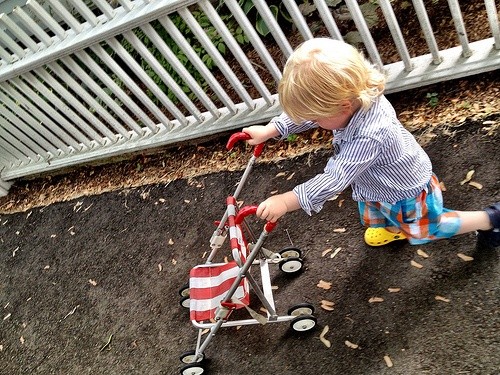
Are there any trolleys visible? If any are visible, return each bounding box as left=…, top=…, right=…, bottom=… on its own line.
left=179, top=131, right=318, bottom=375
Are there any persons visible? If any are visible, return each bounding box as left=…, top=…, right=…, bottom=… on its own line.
left=242, top=37, right=500, bottom=249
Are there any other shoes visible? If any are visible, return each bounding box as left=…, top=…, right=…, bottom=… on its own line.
left=475, top=202, right=500, bottom=251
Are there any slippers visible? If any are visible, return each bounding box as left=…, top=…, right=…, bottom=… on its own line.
left=364, top=226, right=407, bottom=246
